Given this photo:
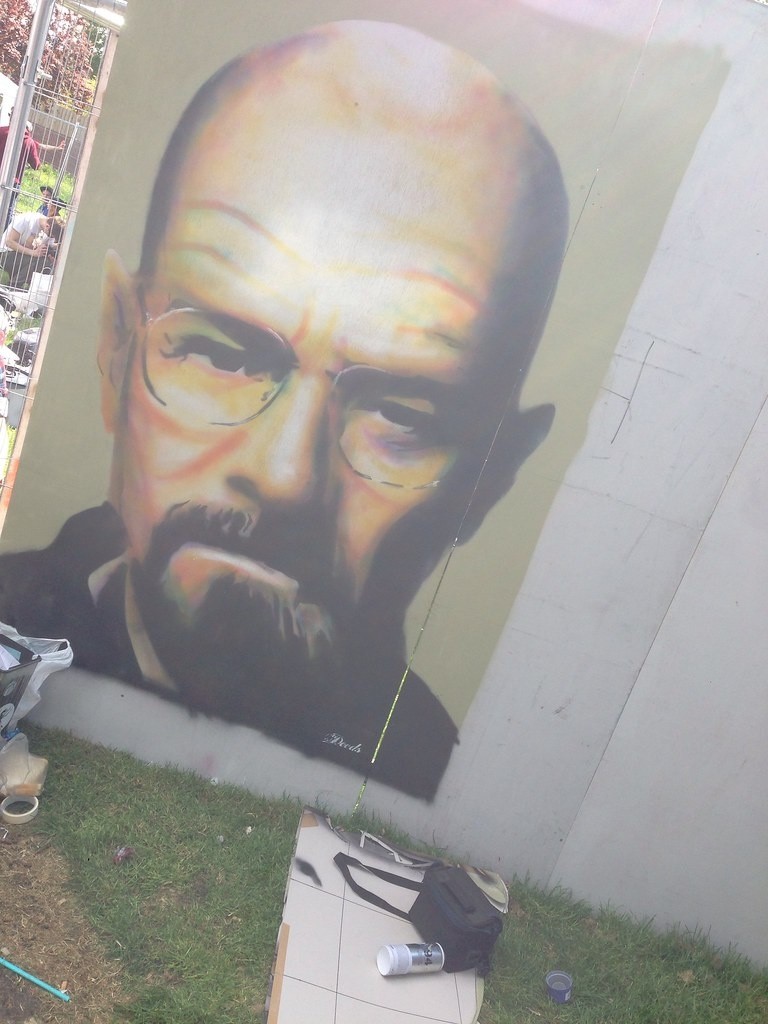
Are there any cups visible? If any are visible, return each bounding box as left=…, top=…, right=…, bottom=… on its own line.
left=545, top=970, right=573, bottom=1003
left=377, top=941, right=445, bottom=976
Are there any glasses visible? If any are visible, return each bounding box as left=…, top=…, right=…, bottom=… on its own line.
left=137, top=285, right=522, bottom=489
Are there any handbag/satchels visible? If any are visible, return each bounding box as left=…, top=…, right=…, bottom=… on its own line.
left=333, top=850, right=503, bottom=973
left=27, top=267, right=54, bottom=315
left=0, top=621, right=76, bottom=733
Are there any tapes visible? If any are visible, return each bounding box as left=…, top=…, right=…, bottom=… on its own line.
left=0, top=794, right=39, bottom=825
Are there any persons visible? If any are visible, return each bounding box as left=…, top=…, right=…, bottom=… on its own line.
left=0, top=305, right=9, bottom=490
left=0, top=106, right=66, bottom=313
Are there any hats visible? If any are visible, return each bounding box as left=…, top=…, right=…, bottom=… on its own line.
left=26, top=121, right=32, bottom=133
left=40, top=186, right=53, bottom=194
left=51, top=197, right=67, bottom=208
